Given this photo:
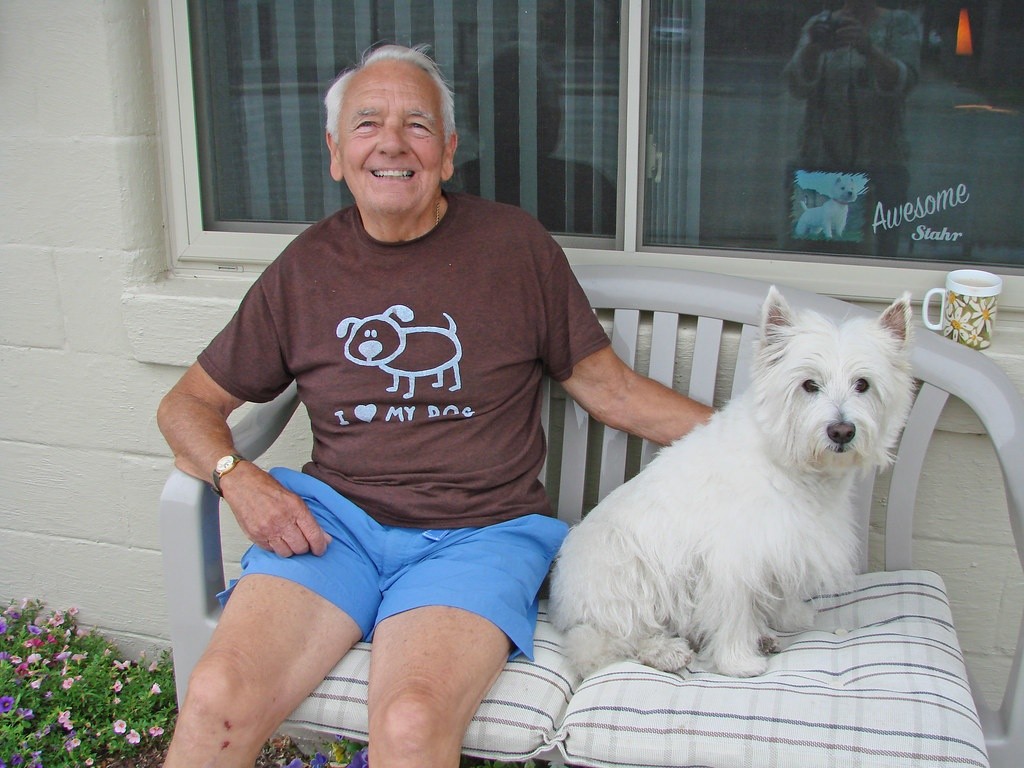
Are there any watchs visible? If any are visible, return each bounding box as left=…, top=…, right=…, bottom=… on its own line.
left=211, top=453, right=248, bottom=496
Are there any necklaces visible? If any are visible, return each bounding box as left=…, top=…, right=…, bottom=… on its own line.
left=435, top=203, right=440, bottom=225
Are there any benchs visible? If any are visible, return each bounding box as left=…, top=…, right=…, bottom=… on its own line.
left=159, top=270, right=1023, bottom=768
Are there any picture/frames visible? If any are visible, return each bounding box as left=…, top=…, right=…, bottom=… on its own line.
left=782, top=160, right=883, bottom=257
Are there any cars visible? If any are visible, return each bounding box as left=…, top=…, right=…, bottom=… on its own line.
left=651, top=16, right=693, bottom=53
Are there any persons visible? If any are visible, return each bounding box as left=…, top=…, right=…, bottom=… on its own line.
left=784, top=0, right=921, bottom=257
left=156, top=47, right=721, bottom=768
left=442, top=48, right=617, bottom=237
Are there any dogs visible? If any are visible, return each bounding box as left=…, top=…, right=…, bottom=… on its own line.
left=549, top=288, right=918, bottom=677
left=796, top=177, right=857, bottom=237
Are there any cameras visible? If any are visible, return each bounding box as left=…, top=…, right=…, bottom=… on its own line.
left=812, top=11, right=846, bottom=51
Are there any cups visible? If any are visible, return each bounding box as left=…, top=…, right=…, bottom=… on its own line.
left=922, top=268, right=1003, bottom=350
